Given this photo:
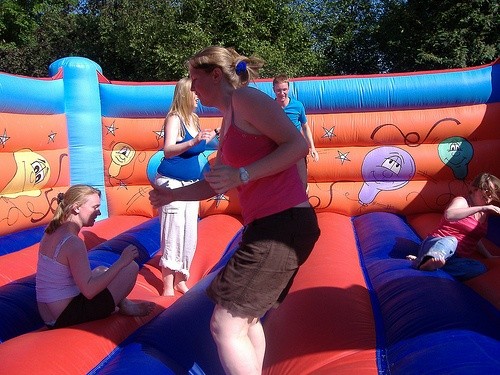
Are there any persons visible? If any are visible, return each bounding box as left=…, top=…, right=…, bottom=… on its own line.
left=405, top=173, right=500, bottom=281
left=154, top=77, right=220, bottom=297
left=272, top=75, right=319, bottom=192
left=148, top=45, right=321, bottom=375
left=35, top=184, right=156, bottom=329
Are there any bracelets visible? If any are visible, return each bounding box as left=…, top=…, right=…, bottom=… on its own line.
left=214, top=128, right=220, bottom=135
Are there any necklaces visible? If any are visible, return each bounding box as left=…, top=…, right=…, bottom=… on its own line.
left=469, top=199, right=483, bottom=222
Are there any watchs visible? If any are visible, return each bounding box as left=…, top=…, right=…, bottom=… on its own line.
left=239, top=166, right=250, bottom=185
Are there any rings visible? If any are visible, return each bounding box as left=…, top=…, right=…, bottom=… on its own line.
left=316, top=159, right=318, bottom=161
